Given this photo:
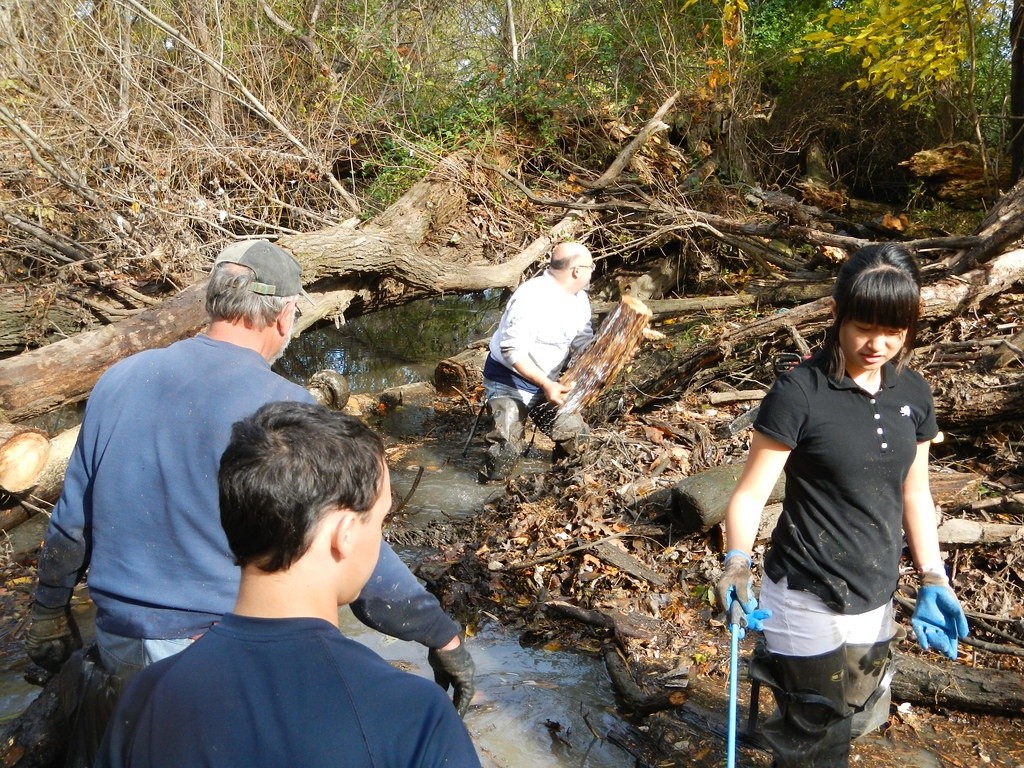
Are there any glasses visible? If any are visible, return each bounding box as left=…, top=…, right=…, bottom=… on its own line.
left=570, top=263, right=596, bottom=272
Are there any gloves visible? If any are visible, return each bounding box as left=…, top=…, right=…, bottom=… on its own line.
left=716, top=550, right=773, bottom=640
left=427, top=643, right=475, bottom=718
left=911, top=573, right=969, bottom=661
left=24, top=620, right=82, bottom=675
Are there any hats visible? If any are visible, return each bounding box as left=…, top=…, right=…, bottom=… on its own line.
left=212, top=238, right=315, bottom=305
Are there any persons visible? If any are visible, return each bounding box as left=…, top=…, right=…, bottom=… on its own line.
left=718, top=243, right=969, bottom=768
left=22, top=239, right=476, bottom=749
left=95, top=402, right=482, bottom=767
left=478, top=241, right=598, bottom=488
